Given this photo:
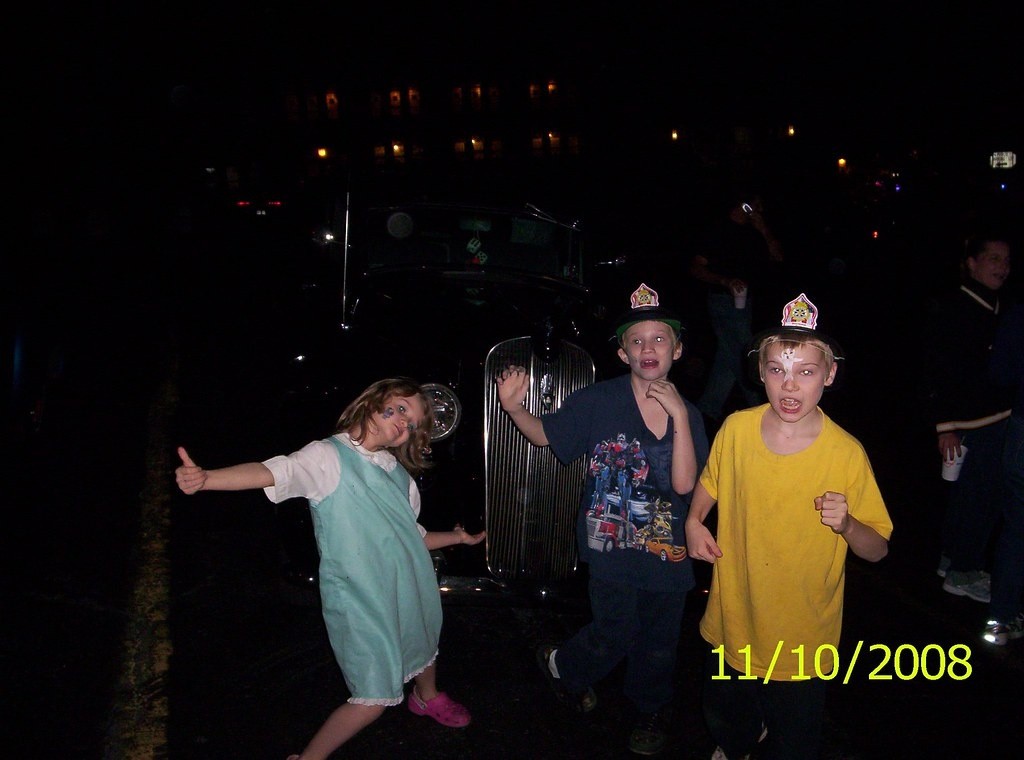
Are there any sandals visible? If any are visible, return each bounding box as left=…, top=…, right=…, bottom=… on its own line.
left=408, top=684, right=472, bottom=728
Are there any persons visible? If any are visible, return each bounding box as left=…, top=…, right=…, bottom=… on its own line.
left=690, top=198, right=786, bottom=430
left=682, top=288, right=893, bottom=760
left=494, top=279, right=709, bottom=756
left=175, top=379, right=487, bottom=760
left=910, top=222, right=1024, bottom=645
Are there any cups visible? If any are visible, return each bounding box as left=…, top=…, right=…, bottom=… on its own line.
left=941, top=445, right=968, bottom=481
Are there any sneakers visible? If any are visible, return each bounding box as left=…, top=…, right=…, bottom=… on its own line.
left=943, top=568, right=992, bottom=603
left=982, top=612, right=1024, bottom=647
left=937, top=552, right=953, bottom=577
left=710, top=721, right=768, bottom=760
left=629, top=706, right=673, bottom=755
left=531, top=643, right=597, bottom=713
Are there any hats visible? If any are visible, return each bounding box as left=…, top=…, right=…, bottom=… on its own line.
left=752, top=284, right=845, bottom=372
left=614, top=283, right=681, bottom=348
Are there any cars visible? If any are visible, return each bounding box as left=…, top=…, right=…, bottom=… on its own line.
left=289, top=184, right=704, bottom=632
